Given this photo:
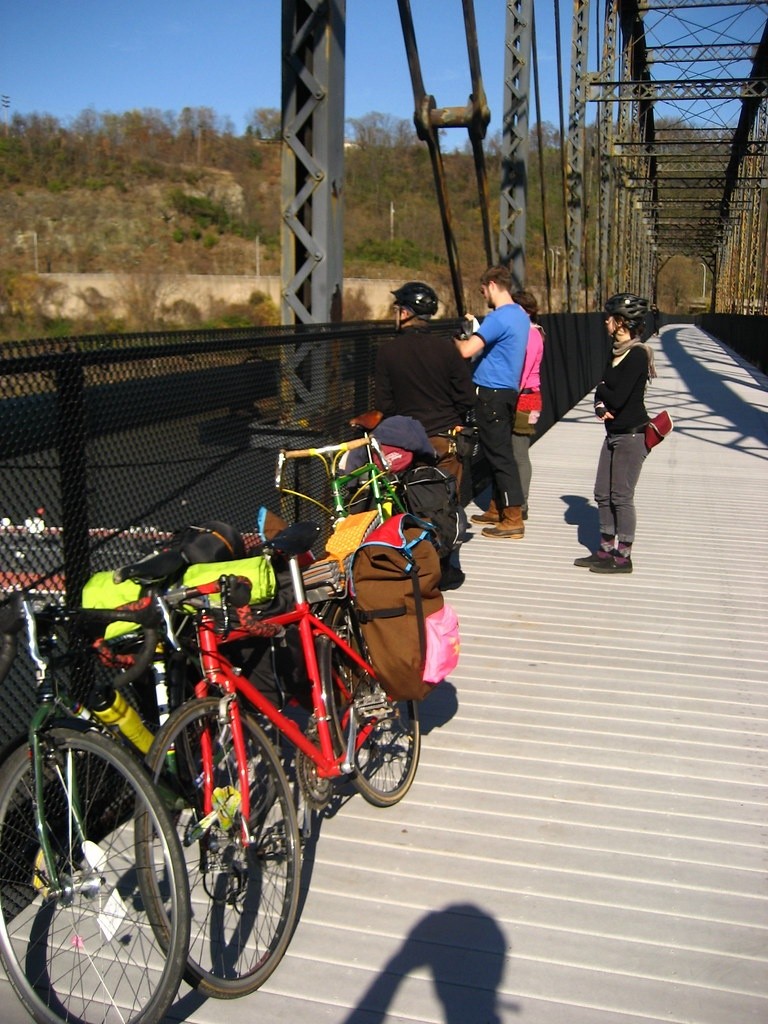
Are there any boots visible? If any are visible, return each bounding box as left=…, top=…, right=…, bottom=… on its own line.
left=471, top=499, right=501, bottom=525
left=481, top=506, right=525, bottom=539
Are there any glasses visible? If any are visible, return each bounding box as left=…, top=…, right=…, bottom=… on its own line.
left=478, top=288, right=484, bottom=294
left=392, top=301, right=399, bottom=313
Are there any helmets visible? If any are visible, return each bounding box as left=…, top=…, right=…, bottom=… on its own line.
left=390, top=282, right=439, bottom=315
left=604, top=293, right=649, bottom=329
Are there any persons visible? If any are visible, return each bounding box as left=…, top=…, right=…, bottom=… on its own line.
left=574, top=293, right=656, bottom=573
left=652, top=304, right=659, bottom=335
left=374, top=282, right=475, bottom=591
left=452, top=265, right=545, bottom=538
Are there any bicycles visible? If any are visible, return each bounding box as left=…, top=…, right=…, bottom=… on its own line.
left=273, top=410, right=470, bottom=590
left=0, top=553, right=284, bottom=1023
left=80, top=519, right=422, bottom=1001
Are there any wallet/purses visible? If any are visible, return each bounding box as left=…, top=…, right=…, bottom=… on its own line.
left=646, top=410, right=674, bottom=450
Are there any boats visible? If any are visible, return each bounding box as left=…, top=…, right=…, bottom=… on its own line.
left=1, top=519, right=263, bottom=600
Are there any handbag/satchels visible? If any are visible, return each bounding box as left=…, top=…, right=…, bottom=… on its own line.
left=350, top=515, right=462, bottom=700
left=399, top=462, right=465, bottom=565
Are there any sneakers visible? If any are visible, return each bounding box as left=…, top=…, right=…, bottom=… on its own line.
left=574, top=553, right=615, bottom=567
left=590, top=557, right=633, bottom=573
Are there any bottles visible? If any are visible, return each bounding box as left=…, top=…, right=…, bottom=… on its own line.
left=90, top=686, right=155, bottom=761
